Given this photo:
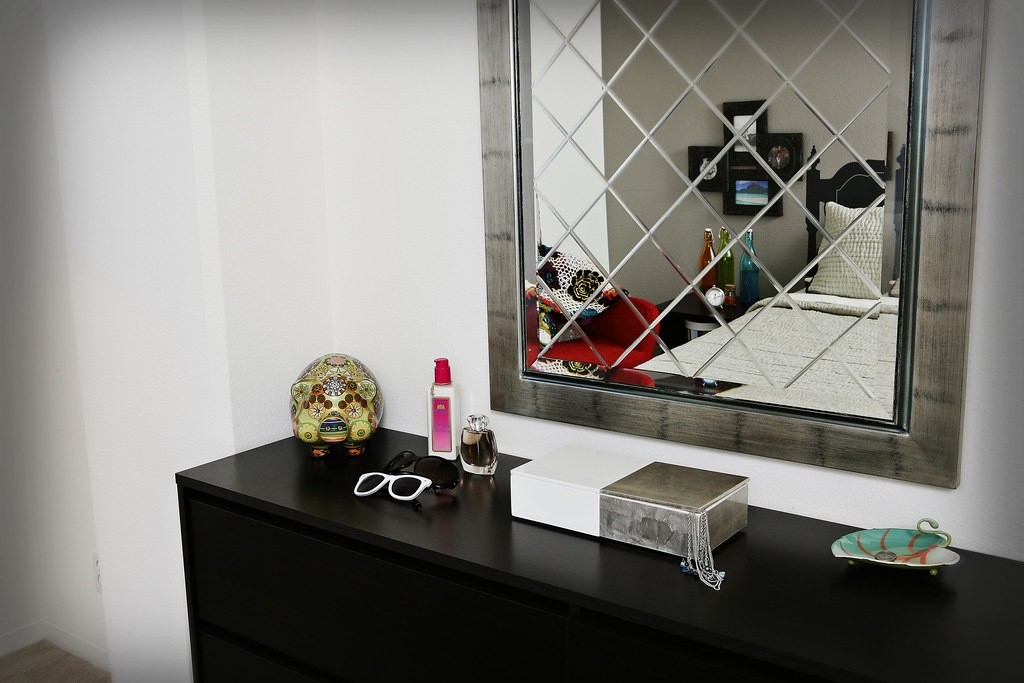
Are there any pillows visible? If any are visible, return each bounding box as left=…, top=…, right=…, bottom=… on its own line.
left=806, top=195, right=889, bottom=302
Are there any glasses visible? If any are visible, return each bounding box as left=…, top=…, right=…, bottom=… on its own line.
left=353, top=472, right=432, bottom=510
left=382, top=451, right=461, bottom=495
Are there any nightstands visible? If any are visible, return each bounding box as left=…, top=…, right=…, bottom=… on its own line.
left=653, top=288, right=762, bottom=351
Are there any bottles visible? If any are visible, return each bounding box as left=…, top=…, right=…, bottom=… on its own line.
left=699, top=228, right=717, bottom=294
left=724, top=284, right=736, bottom=306
left=739, top=229, right=760, bottom=304
left=717, top=226, right=735, bottom=296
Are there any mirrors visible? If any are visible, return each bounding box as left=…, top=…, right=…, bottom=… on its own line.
left=515, top=0, right=923, bottom=438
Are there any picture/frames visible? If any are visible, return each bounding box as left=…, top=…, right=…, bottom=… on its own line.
left=686, top=99, right=806, bottom=217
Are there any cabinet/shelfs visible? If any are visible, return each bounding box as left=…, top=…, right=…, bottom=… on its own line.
left=174, top=427, right=1019, bottom=683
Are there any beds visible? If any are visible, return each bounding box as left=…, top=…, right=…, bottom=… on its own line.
left=635, top=284, right=899, bottom=424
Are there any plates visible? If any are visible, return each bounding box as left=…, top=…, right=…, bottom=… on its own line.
left=831, top=518, right=961, bottom=575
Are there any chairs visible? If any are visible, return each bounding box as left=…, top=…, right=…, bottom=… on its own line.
left=523, top=287, right=662, bottom=369
